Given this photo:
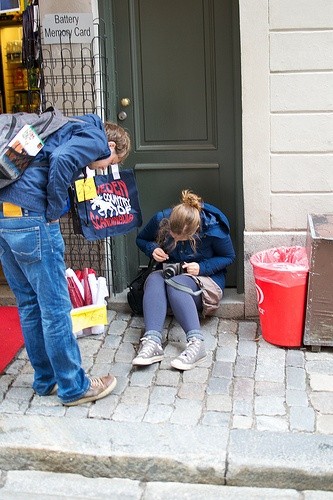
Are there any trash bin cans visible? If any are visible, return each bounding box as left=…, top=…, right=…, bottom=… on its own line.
left=250, top=247, right=309, bottom=349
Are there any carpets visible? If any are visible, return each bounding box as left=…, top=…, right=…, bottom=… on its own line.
left=0, top=304, right=25, bottom=376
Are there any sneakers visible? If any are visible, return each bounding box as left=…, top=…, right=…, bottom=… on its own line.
left=132, top=336, right=165, bottom=366
left=170, top=337, right=208, bottom=370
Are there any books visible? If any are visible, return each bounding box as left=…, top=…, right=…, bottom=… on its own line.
left=0, top=124, right=45, bottom=181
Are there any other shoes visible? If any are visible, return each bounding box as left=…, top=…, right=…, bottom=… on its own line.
left=50, top=375, right=117, bottom=406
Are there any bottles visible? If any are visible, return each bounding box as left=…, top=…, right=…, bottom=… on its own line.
left=5, top=39, right=41, bottom=117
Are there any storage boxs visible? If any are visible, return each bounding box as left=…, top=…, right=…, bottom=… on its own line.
left=70, top=300, right=107, bottom=339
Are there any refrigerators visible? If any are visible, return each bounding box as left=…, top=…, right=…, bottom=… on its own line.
left=0, top=10, right=43, bottom=117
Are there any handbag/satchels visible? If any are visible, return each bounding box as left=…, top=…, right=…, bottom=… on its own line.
left=127, top=267, right=151, bottom=317
left=67, top=164, right=143, bottom=242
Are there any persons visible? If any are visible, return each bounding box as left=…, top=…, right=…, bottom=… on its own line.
left=0, top=114, right=130, bottom=406
left=131, top=193, right=236, bottom=371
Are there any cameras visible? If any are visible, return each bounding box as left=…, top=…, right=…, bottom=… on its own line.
left=162, top=263, right=181, bottom=279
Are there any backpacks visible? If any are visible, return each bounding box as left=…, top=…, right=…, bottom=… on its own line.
left=0, top=105, right=102, bottom=190
